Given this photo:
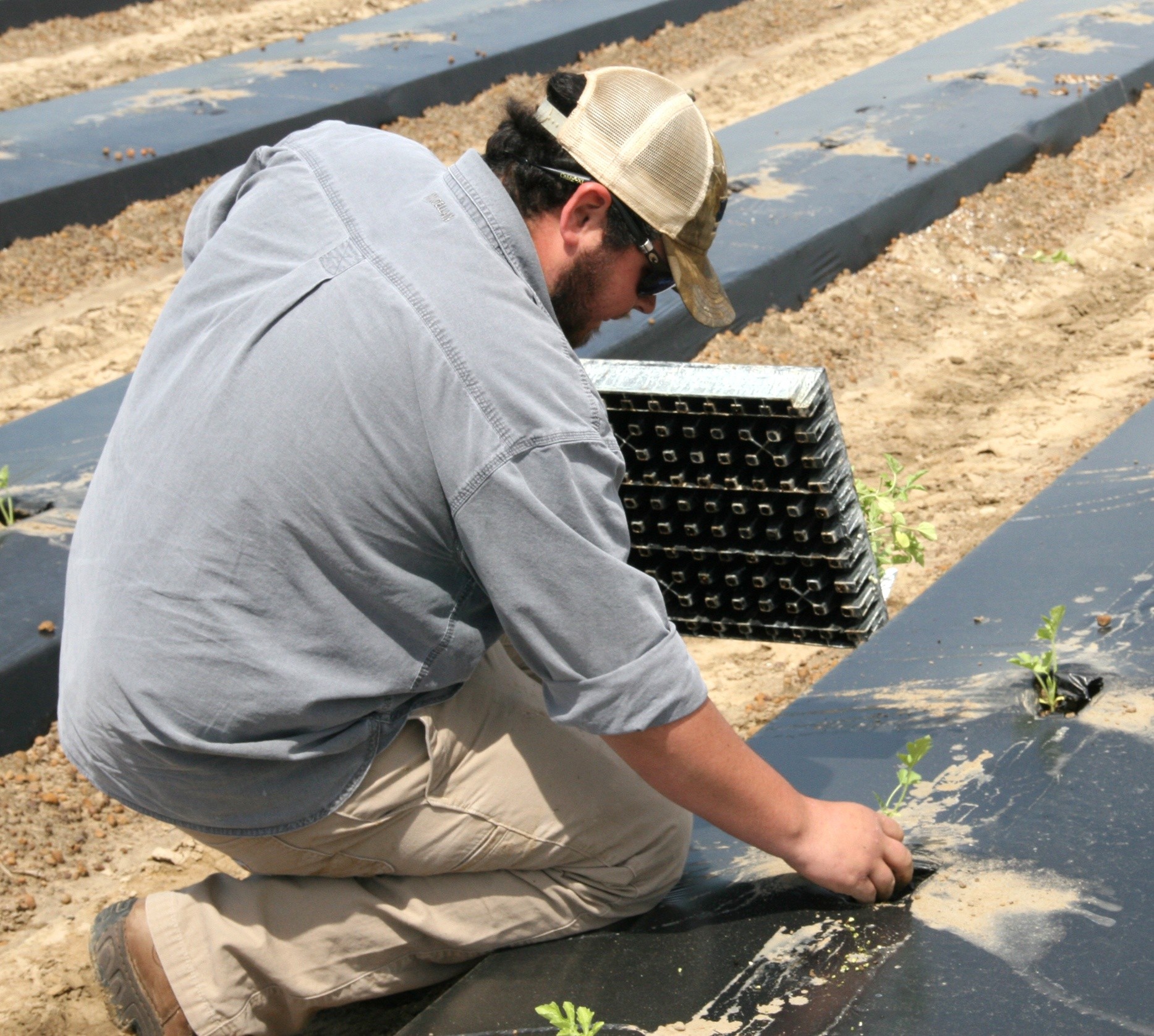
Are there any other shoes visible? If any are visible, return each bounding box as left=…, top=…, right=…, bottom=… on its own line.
left=91, top=894, right=193, bottom=1036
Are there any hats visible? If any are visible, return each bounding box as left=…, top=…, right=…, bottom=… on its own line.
left=533, top=65, right=736, bottom=328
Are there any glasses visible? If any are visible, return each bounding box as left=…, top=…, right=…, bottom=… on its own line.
left=510, top=150, right=679, bottom=299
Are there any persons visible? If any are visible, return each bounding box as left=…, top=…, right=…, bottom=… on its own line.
left=55, top=62, right=914, bottom=1036
